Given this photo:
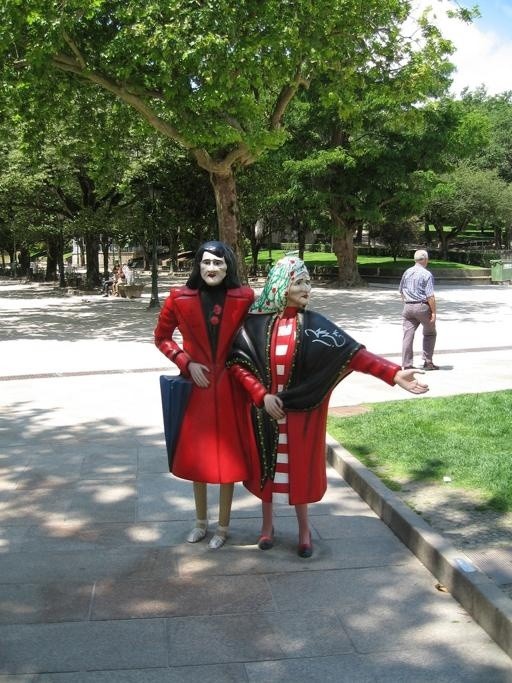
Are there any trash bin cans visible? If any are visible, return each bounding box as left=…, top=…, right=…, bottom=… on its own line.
left=490, top=259, right=512, bottom=285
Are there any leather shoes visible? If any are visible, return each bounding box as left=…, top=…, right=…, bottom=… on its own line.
left=297, top=530, right=313, bottom=558
left=258, top=524, right=275, bottom=550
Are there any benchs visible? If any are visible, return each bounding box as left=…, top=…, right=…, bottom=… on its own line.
left=107, top=284, right=144, bottom=298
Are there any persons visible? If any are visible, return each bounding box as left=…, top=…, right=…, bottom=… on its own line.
left=225, top=256, right=429, bottom=558
left=101, top=264, right=135, bottom=297
left=399, top=250, right=440, bottom=370
left=155, top=240, right=254, bottom=550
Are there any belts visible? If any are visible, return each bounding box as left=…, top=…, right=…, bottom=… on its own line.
left=406, top=302, right=428, bottom=304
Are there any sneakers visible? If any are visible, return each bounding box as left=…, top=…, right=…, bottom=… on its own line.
left=404, top=361, right=439, bottom=370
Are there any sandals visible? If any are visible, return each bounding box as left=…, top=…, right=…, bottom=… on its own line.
left=186, top=519, right=229, bottom=549
